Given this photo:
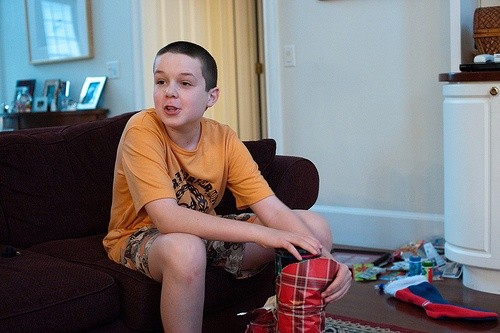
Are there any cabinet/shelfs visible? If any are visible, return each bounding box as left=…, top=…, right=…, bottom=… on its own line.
left=437, top=71, right=500, bottom=296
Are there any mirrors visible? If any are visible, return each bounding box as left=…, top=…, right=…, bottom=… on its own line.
left=25, top=0, right=95, bottom=65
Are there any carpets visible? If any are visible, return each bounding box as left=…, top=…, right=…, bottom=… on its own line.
left=322, top=254, right=399, bottom=332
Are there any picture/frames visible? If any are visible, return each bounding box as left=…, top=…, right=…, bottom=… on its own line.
left=15, top=77, right=108, bottom=110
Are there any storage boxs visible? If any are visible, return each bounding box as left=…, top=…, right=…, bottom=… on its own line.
left=474, top=4, right=500, bottom=54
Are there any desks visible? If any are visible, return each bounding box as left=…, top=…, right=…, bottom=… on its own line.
left=326, top=276, right=500, bottom=332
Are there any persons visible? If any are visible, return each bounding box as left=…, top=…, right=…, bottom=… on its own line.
left=101, top=41, right=353, bottom=333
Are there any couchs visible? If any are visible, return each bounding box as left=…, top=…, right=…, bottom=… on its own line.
left=0, top=110, right=320, bottom=332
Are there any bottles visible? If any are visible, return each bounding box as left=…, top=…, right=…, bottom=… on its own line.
left=409, top=256, right=421, bottom=277
left=421, top=261, right=433, bottom=284
